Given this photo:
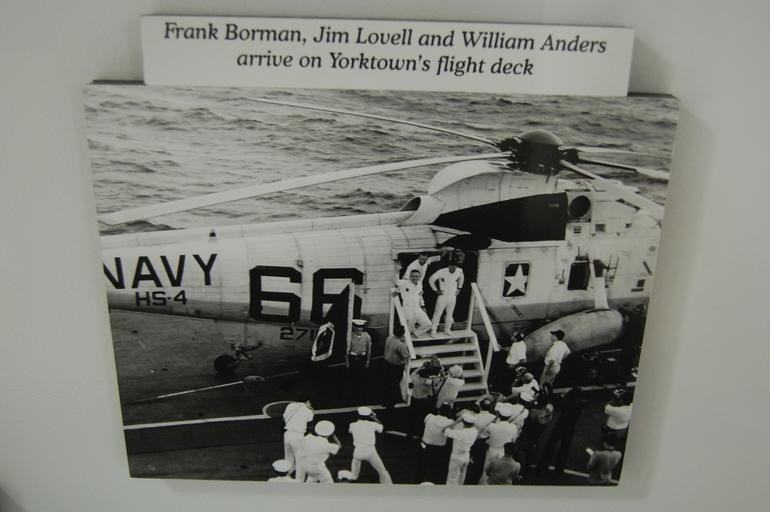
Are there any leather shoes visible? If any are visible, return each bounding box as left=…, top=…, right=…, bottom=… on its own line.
left=430, top=328, right=453, bottom=337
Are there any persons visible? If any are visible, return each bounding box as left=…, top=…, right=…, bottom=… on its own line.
left=393, top=265, right=433, bottom=338
left=392, top=252, right=447, bottom=312
left=428, top=261, right=464, bottom=337
left=266, top=318, right=634, bottom=486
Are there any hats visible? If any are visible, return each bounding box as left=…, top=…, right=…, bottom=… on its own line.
left=550, top=329, right=564, bottom=340
left=357, top=407, right=373, bottom=416
left=462, top=414, right=476, bottom=423
left=449, top=365, right=462, bottom=378
left=497, top=403, right=513, bottom=416
left=272, top=459, right=292, bottom=473
left=352, top=318, right=368, bottom=325
left=314, top=420, right=335, bottom=436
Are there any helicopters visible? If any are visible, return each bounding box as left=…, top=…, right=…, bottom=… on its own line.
left=96, top=93, right=671, bottom=405
